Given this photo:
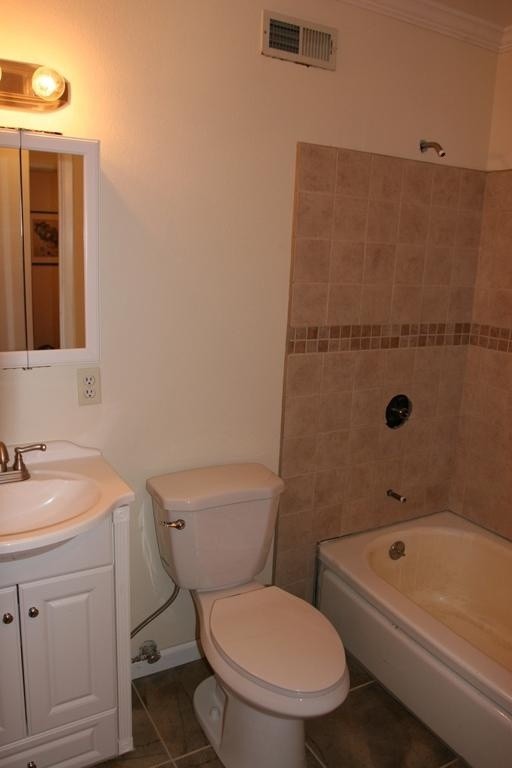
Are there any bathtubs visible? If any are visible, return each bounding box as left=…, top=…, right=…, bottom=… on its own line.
left=317, top=510, right=512, bottom=768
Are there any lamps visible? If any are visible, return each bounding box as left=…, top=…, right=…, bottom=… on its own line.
left=2, top=59, right=73, bottom=114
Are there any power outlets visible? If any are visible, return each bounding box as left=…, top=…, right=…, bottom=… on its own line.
left=77, top=366, right=101, bottom=406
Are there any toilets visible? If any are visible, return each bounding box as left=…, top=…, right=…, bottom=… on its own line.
left=144, top=462, right=351, bottom=768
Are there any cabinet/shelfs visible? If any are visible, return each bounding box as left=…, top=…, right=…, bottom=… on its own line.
left=0, top=505, right=139, bottom=768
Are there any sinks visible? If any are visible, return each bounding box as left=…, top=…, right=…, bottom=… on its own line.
left=0, top=477, right=101, bottom=536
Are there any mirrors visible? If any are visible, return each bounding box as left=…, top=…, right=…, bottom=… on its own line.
left=0, top=125, right=102, bottom=367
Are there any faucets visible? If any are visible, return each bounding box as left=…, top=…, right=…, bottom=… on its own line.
left=0, top=440, right=9, bottom=473
